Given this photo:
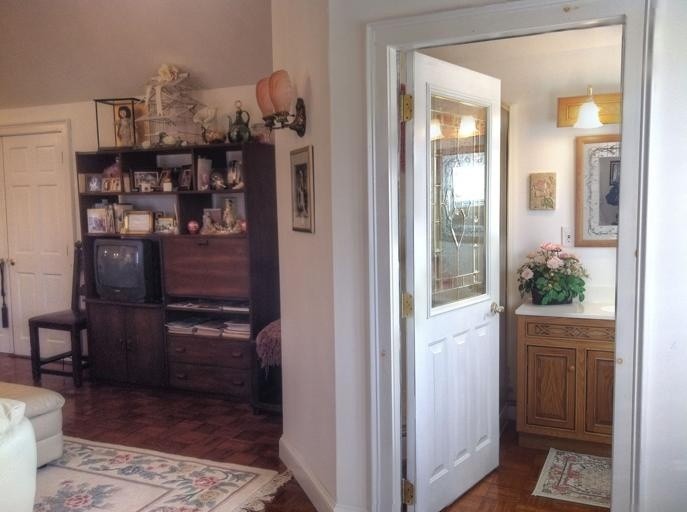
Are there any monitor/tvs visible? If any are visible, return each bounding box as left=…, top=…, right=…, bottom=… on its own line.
left=93, top=238, right=160, bottom=303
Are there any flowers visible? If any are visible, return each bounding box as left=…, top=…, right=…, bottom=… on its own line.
left=518, top=242, right=589, bottom=305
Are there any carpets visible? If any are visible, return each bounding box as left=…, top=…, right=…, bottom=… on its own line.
left=36, top=435, right=292, bottom=512
left=532, top=448, right=611, bottom=508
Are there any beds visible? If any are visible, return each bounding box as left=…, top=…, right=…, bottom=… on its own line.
left=1, top=382, right=65, bottom=469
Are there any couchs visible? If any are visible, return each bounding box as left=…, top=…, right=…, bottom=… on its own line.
left=1, top=398, right=37, bottom=512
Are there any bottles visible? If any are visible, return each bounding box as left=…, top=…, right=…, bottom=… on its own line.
left=162, top=177, right=172, bottom=192
left=223, top=198, right=236, bottom=228
left=252, top=122, right=269, bottom=144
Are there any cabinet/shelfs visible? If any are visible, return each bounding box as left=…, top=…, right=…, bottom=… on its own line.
left=514, top=301, right=615, bottom=445
left=75, top=143, right=279, bottom=414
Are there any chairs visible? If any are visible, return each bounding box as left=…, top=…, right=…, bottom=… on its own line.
left=29, top=240, right=89, bottom=388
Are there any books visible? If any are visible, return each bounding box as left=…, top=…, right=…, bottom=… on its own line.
left=164, top=316, right=251, bottom=339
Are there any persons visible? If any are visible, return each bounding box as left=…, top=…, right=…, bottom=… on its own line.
left=605, top=176, right=619, bottom=207
left=115, top=105, right=138, bottom=145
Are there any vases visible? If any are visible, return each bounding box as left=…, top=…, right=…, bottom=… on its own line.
left=531, top=286, right=572, bottom=305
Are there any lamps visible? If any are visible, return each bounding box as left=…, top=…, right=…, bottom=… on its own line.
left=256, top=68, right=305, bottom=137
left=574, top=86, right=603, bottom=129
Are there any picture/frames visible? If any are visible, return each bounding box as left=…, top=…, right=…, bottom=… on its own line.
left=290, top=145, right=314, bottom=233
left=575, top=135, right=621, bottom=247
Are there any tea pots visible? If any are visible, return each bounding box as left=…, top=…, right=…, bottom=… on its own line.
left=226, top=107, right=251, bottom=143
